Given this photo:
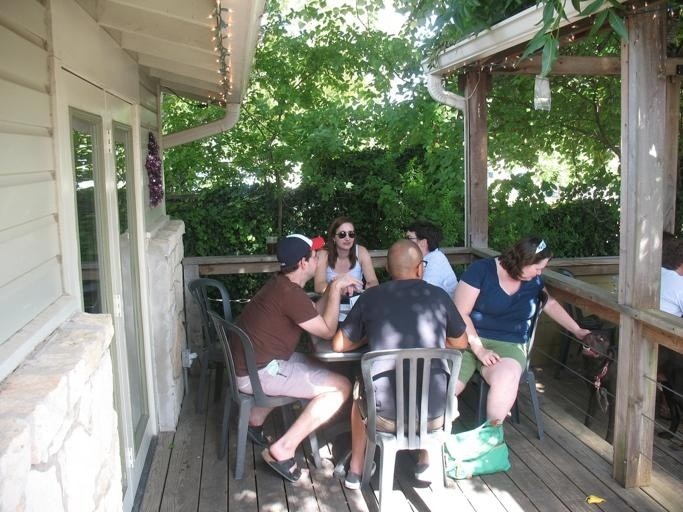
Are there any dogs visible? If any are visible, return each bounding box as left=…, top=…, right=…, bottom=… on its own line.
left=579, top=328, right=683, bottom=444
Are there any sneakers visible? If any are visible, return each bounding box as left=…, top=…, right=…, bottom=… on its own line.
left=413, top=463, right=433, bottom=483
left=343, top=460, right=377, bottom=490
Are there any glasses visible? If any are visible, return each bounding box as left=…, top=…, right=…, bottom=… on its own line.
left=335, top=231, right=356, bottom=239
left=404, top=235, right=419, bottom=241
left=417, top=260, right=428, bottom=269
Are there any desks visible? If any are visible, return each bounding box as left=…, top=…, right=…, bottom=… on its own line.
left=307, top=300, right=367, bottom=363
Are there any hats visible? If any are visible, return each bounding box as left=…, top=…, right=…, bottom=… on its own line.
left=275, top=233, right=325, bottom=268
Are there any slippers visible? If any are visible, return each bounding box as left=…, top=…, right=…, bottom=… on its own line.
left=261, top=448, right=303, bottom=483
left=234, top=415, right=273, bottom=448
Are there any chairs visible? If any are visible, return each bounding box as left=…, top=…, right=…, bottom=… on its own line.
left=187, top=278, right=235, bottom=415
left=469, top=290, right=548, bottom=441
left=207, top=309, right=321, bottom=481
left=556, top=267, right=615, bottom=378
left=359, top=346, right=463, bottom=512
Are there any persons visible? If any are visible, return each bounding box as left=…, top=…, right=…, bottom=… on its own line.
left=406, top=221, right=458, bottom=298
left=226, top=233, right=353, bottom=482
left=313, top=218, right=381, bottom=296
left=659, top=229, right=683, bottom=317
left=332, top=240, right=468, bottom=490
left=448, top=233, right=590, bottom=431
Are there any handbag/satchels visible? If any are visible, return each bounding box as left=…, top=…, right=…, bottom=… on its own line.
left=440, top=416, right=511, bottom=481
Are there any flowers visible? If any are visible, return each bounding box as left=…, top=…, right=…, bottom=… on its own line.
left=145, top=132, right=164, bottom=208
